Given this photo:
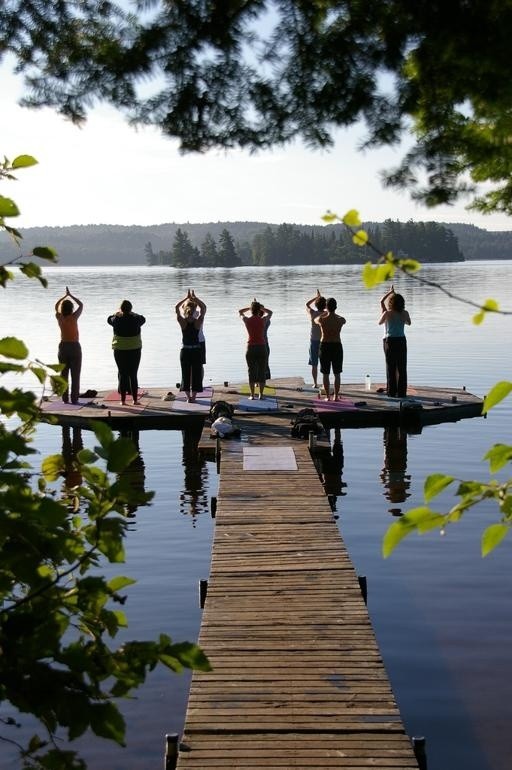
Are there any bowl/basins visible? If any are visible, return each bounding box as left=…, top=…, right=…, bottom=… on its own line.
left=163, top=395, right=176, bottom=401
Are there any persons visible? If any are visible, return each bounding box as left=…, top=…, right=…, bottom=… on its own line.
left=378, top=294, right=411, bottom=397
left=179, top=428, right=207, bottom=515
left=58, top=426, right=85, bottom=513
left=238, top=297, right=273, bottom=400
left=377, top=285, right=407, bottom=393
left=314, top=298, right=346, bottom=403
left=182, top=303, right=206, bottom=387
left=117, top=370, right=138, bottom=394
left=55, top=286, right=83, bottom=403
left=386, top=429, right=410, bottom=515
left=380, top=430, right=395, bottom=488
left=115, top=430, right=154, bottom=518
left=304, top=289, right=327, bottom=388
left=106, top=300, right=146, bottom=406
left=315, top=428, right=345, bottom=514
left=175, top=290, right=206, bottom=404
left=253, top=304, right=271, bottom=387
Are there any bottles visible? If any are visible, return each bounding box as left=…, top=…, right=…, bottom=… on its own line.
left=365, top=374, right=371, bottom=390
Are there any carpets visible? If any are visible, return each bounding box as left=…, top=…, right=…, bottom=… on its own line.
left=243, top=447, right=298, bottom=471
